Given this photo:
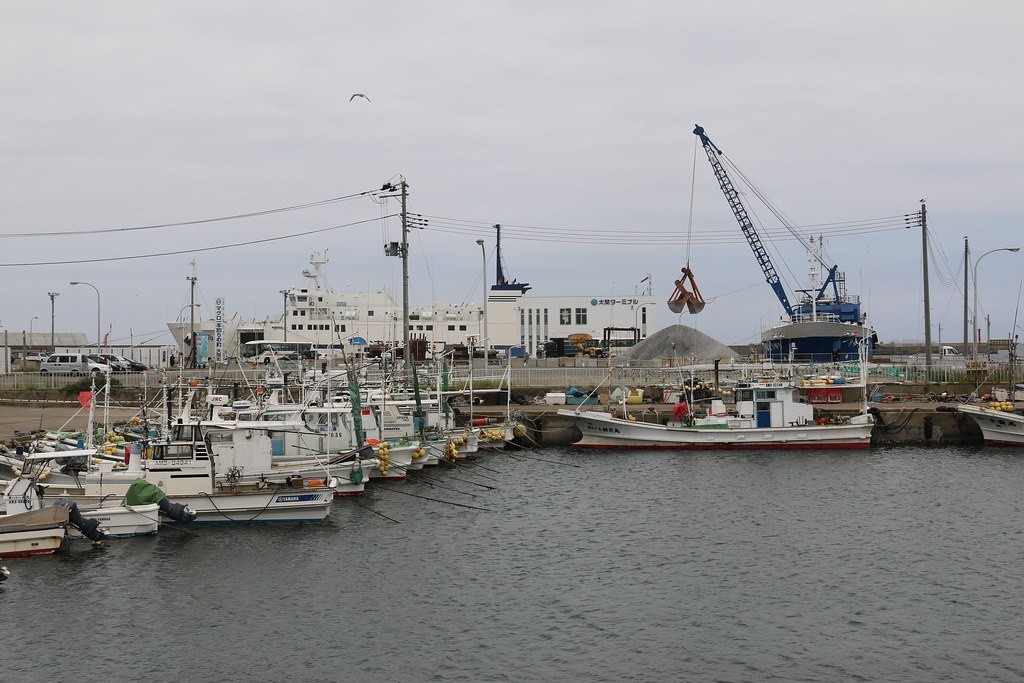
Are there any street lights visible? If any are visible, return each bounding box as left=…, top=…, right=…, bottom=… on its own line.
left=48, top=292, right=60, bottom=353
left=973, top=248, right=1021, bottom=361
left=29, top=317, right=38, bottom=349
left=71, top=281, right=101, bottom=355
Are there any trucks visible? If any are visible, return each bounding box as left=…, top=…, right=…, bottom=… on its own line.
left=911, top=346, right=959, bottom=359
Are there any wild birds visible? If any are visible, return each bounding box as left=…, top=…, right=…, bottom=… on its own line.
left=350, top=94, right=371, bottom=103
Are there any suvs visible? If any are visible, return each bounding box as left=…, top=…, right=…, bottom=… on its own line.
left=241, top=340, right=315, bottom=364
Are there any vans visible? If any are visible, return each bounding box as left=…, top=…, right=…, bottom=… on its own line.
left=100, top=354, right=131, bottom=372
left=40, top=353, right=88, bottom=375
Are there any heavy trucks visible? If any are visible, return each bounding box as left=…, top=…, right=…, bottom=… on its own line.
left=536, top=336, right=599, bottom=358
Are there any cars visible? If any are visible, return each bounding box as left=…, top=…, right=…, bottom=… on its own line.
left=88, top=358, right=113, bottom=375
left=122, top=356, right=148, bottom=374
left=88, top=356, right=117, bottom=372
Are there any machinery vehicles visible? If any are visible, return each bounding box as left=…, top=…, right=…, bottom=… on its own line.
left=568, top=333, right=604, bottom=359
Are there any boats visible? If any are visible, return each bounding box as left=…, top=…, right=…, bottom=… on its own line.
left=958, top=343, right=1024, bottom=444
left=668, top=125, right=878, bottom=362
left=557, top=374, right=875, bottom=450
left=167, top=223, right=656, bottom=362
left=0, top=319, right=512, bottom=559
